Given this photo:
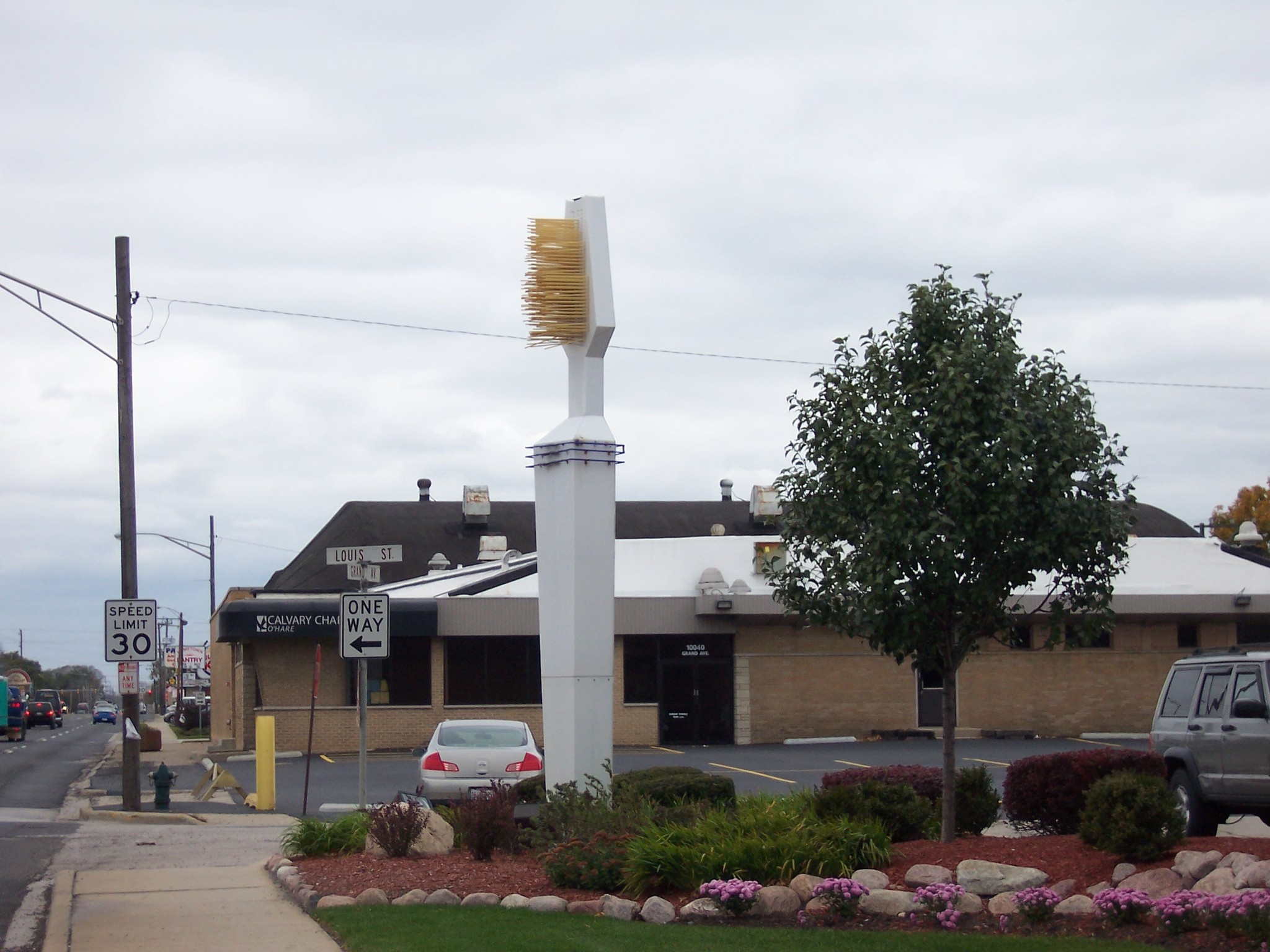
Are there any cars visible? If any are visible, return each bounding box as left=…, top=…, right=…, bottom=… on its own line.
left=26, top=701, right=58, bottom=730
left=75, top=698, right=123, bottom=725
left=411, top=719, right=545, bottom=807
left=140, top=702, right=147, bottom=714
left=61, top=701, right=69, bottom=714
left=163, top=696, right=211, bottom=730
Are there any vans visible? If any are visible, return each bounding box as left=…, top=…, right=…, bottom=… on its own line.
left=30, top=689, right=65, bottom=728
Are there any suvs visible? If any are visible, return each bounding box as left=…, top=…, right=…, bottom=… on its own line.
left=0, top=686, right=30, bottom=742
left=1149, top=643, right=1269, bottom=837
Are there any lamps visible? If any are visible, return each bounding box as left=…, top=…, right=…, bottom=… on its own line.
left=717, top=600, right=732, bottom=609
left=1234, top=587, right=1251, bottom=606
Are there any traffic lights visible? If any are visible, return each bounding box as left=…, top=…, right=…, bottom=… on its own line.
left=149, top=691, right=152, bottom=696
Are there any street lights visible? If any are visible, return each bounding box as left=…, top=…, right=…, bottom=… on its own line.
left=156, top=606, right=183, bottom=726
left=114, top=531, right=216, bottom=615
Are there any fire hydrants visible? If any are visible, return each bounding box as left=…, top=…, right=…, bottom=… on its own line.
left=146, top=761, right=179, bottom=813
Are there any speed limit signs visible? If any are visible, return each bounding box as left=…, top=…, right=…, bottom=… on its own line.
left=103, top=599, right=157, bottom=662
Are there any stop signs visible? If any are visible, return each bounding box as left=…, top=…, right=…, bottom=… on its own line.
left=313, top=644, right=322, bottom=699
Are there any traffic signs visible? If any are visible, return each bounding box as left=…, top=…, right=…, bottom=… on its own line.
left=340, top=592, right=390, bottom=660
left=196, top=691, right=205, bottom=705
